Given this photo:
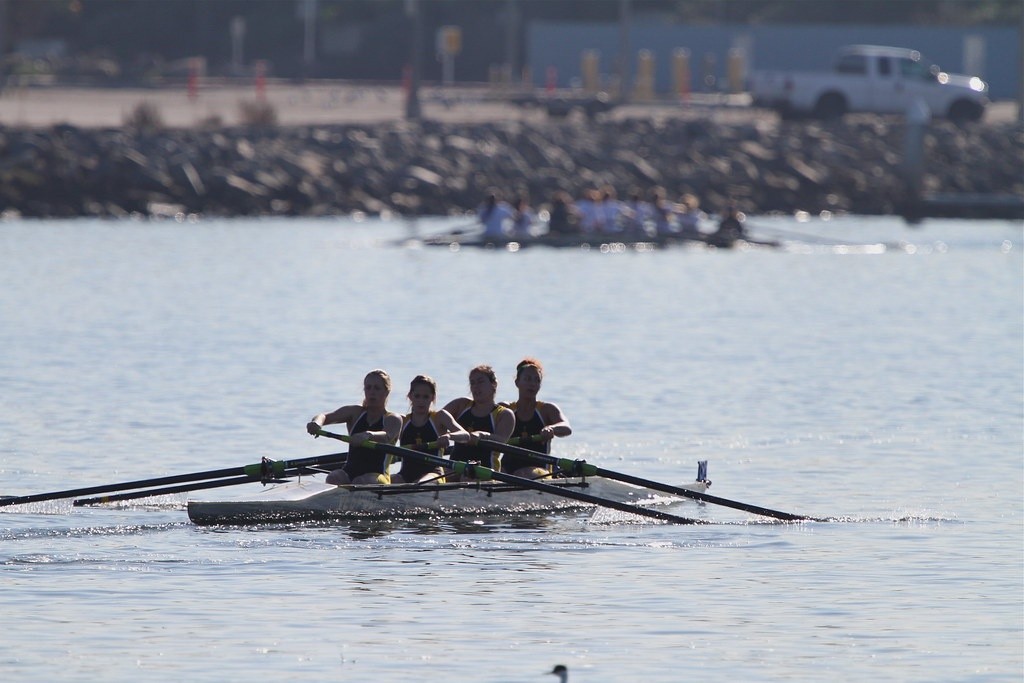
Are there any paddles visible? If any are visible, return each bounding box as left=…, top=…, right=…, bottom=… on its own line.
left=0, top=439, right=449, bottom=508
left=475, top=432, right=826, bottom=526
left=314, top=428, right=712, bottom=525
left=70, top=435, right=550, bottom=508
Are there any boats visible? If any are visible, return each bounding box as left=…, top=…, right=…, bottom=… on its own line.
left=425, top=223, right=748, bottom=254
left=186, top=459, right=713, bottom=523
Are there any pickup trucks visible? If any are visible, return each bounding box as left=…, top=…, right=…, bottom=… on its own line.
left=748, top=40, right=991, bottom=129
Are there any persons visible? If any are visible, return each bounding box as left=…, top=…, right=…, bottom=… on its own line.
left=306, top=360, right=572, bottom=486
left=482, top=184, right=751, bottom=242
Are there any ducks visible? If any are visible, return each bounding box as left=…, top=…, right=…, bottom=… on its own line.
left=547, top=664, right=568, bottom=683
